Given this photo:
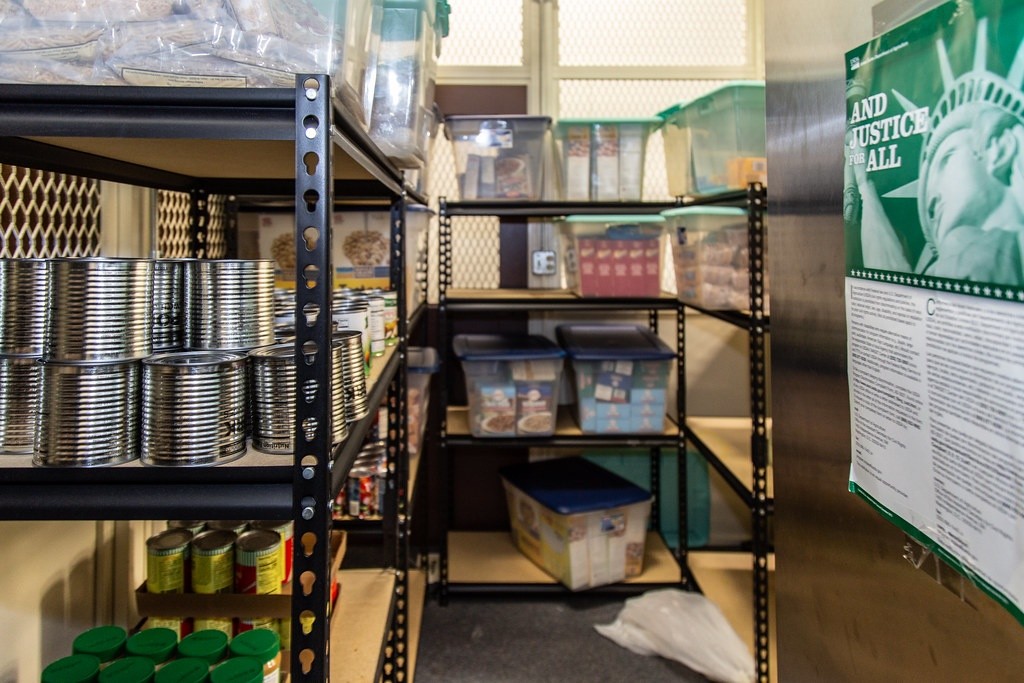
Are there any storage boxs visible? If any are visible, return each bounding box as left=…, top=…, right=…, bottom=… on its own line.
left=554, top=214, right=668, bottom=297
left=551, top=117, right=663, bottom=202
left=655, top=101, right=688, bottom=197
left=453, top=334, right=565, bottom=439
left=555, top=322, right=677, bottom=434
left=680, top=80, right=767, bottom=195
left=0, top=0, right=385, bottom=133
left=373, top=346, right=441, bottom=454
left=660, top=205, right=770, bottom=310
left=367, top=0, right=452, bottom=170
left=500, top=454, right=656, bottom=591
left=443, top=115, right=553, bottom=201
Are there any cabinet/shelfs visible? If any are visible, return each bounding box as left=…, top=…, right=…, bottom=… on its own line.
left=0, top=73, right=767, bottom=683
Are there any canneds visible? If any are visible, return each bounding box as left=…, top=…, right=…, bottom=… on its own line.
left=39, top=518, right=296, bottom=683
left=331, top=439, right=389, bottom=518
left=0, top=255, right=399, bottom=469
